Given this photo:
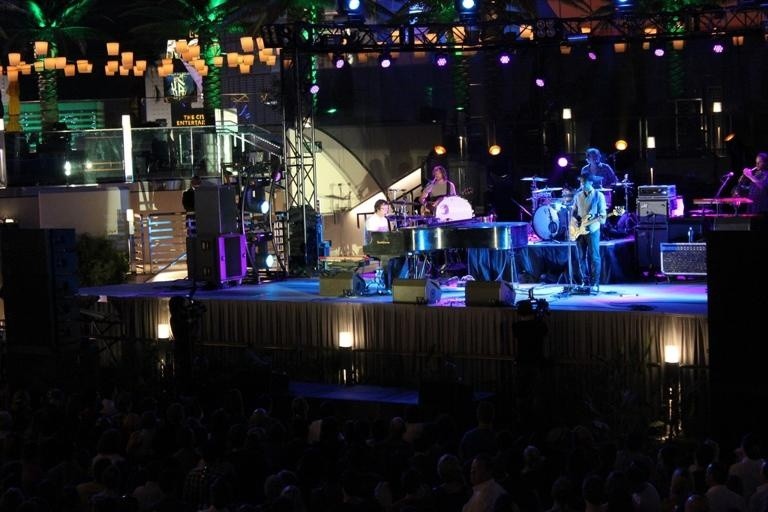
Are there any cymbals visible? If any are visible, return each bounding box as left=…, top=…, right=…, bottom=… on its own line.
left=532, top=187, right=563, bottom=192
left=596, top=188, right=613, bottom=193
left=612, top=182, right=634, bottom=186
left=521, top=176, right=548, bottom=182
left=391, top=200, right=420, bottom=207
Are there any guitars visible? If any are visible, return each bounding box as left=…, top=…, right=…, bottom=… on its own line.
left=569, top=205, right=625, bottom=242
left=420, top=187, right=474, bottom=216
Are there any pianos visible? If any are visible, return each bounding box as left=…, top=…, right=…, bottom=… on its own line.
left=693, top=197, right=754, bottom=206
left=363, top=218, right=528, bottom=283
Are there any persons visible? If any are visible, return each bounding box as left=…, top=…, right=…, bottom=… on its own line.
left=560, top=186, right=573, bottom=202
left=418, top=164, right=457, bottom=216
left=0, top=364, right=768, bottom=512
left=580, top=145, right=618, bottom=212
left=730, top=150, right=767, bottom=217
left=361, top=199, right=408, bottom=292
left=168, top=295, right=210, bottom=389
left=568, top=169, right=607, bottom=295
left=509, top=293, right=558, bottom=425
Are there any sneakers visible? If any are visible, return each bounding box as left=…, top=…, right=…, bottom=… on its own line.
left=591, top=286, right=599, bottom=295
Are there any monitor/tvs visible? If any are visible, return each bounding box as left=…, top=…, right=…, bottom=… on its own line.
left=164, top=72, right=197, bottom=102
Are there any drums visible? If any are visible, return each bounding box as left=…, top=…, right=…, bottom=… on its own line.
left=433, top=195, right=475, bottom=224
left=531, top=201, right=572, bottom=242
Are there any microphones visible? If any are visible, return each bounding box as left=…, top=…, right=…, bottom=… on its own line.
left=433, top=176, right=438, bottom=184
left=724, top=171, right=734, bottom=177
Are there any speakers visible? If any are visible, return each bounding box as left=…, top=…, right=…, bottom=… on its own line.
left=195, top=184, right=236, bottom=235
left=320, top=271, right=366, bottom=297
left=465, top=279, right=516, bottom=308
left=392, top=278, right=442, bottom=304
